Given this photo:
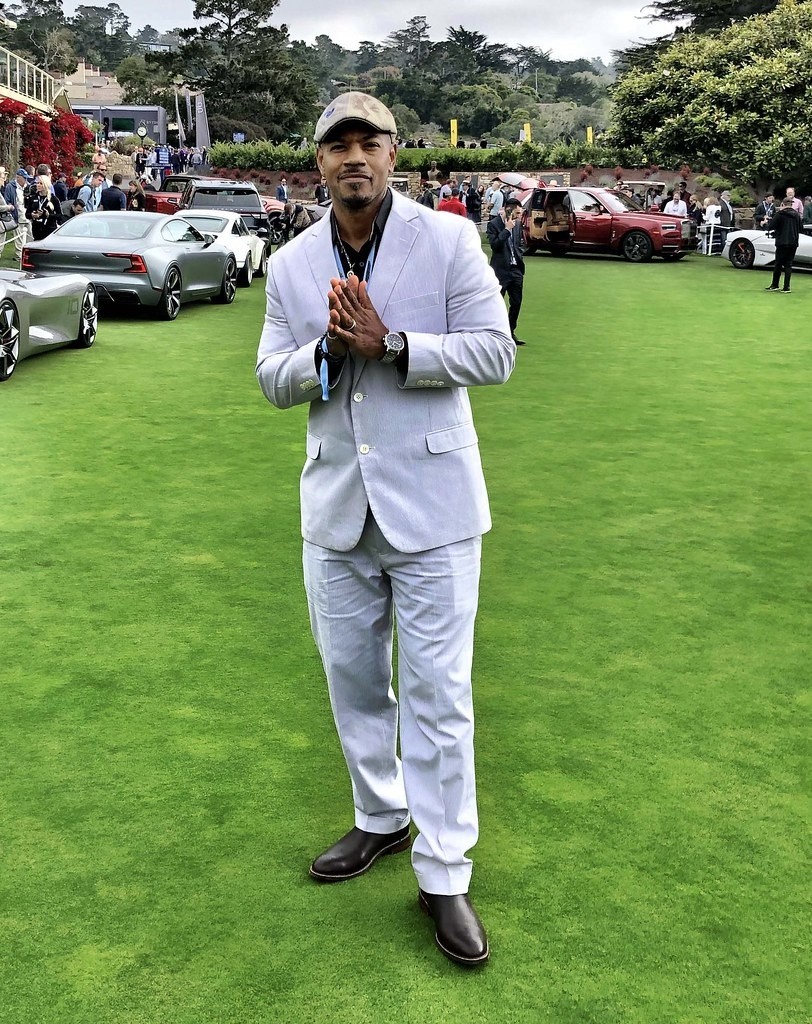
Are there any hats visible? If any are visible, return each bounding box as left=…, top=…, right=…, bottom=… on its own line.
left=284, top=203, right=292, bottom=216
left=16, top=169, right=30, bottom=178
left=443, top=190, right=451, bottom=196
left=190, top=147, right=194, bottom=149
left=58, top=173, right=68, bottom=178
left=783, top=197, right=794, bottom=204
left=0, top=167, right=9, bottom=174
left=314, top=91, right=398, bottom=144
left=546, top=180, right=560, bottom=186
left=721, top=191, right=731, bottom=196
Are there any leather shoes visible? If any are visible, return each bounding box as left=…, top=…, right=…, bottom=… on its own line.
left=513, top=337, right=526, bottom=345
left=309, top=823, right=411, bottom=881
left=418, top=889, right=489, bottom=964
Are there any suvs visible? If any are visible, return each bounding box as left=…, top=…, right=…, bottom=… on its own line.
left=493, top=172, right=698, bottom=262
left=168, top=179, right=272, bottom=258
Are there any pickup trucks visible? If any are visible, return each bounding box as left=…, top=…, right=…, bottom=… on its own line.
left=120, top=176, right=288, bottom=245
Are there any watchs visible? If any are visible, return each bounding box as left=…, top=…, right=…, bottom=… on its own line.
left=378, top=329, right=404, bottom=365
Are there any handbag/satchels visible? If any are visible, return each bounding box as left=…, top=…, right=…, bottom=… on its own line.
left=0, top=211, right=18, bottom=234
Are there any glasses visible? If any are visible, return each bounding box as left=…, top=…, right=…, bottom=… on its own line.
left=617, top=184, right=622, bottom=185
left=99, top=169, right=107, bottom=171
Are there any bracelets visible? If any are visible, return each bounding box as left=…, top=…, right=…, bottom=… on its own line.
left=318, top=336, right=342, bottom=365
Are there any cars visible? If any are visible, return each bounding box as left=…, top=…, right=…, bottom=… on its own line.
left=20, top=210, right=240, bottom=322
left=175, top=206, right=266, bottom=287
left=303, top=197, right=334, bottom=222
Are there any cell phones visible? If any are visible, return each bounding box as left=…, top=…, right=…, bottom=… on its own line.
left=506, top=209, right=512, bottom=222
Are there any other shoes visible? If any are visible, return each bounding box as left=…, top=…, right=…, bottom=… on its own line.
left=765, top=287, right=779, bottom=291
left=780, top=288, right=791, bottom=293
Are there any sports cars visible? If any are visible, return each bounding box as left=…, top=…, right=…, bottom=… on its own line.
left=1, top=265, right=99, bottom=381
left=721, top=224, right=812, bottom=269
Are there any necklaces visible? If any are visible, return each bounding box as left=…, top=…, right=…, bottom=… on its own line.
left=334, top=220, right=354, bottom=277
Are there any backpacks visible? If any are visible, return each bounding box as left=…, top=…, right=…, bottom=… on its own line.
left=67, top=184, right=92, bottom=203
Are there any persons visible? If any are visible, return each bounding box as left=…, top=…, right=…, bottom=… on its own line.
left=509, top=134, right=520, bottom=144
left=555, top=131, right=576, bottom=147
left=718, top=190, right=735, bottom=248
left=418, top=175, right=520, bottom=226
left=132, top=142, right=209, bottom=179
left=275, top=176, right=329, bottom=238
left=0, top=149, right=147, bottom=261
left=596, top=129, right=606, bottom=146
left=276, top=134, right=308, bottom=151
left=487, top=198, right=526, bottom=345
left=613, top=181, right=719, bottom=233
left=764, top=197, right=802, bottom=292
left=428, top=160, right=441, bottom=181
left=398, top=138, right=426, bottom=148
left=753, top=188, right=812, bottom=236
left=256, top=93, right=518, bottom=960
left=456, top=137, right=487, bottom=149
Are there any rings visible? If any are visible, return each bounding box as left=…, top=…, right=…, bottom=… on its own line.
left=346, top=320, right=357, bottom=331
left=327, top=332, right=337, bottom=341
